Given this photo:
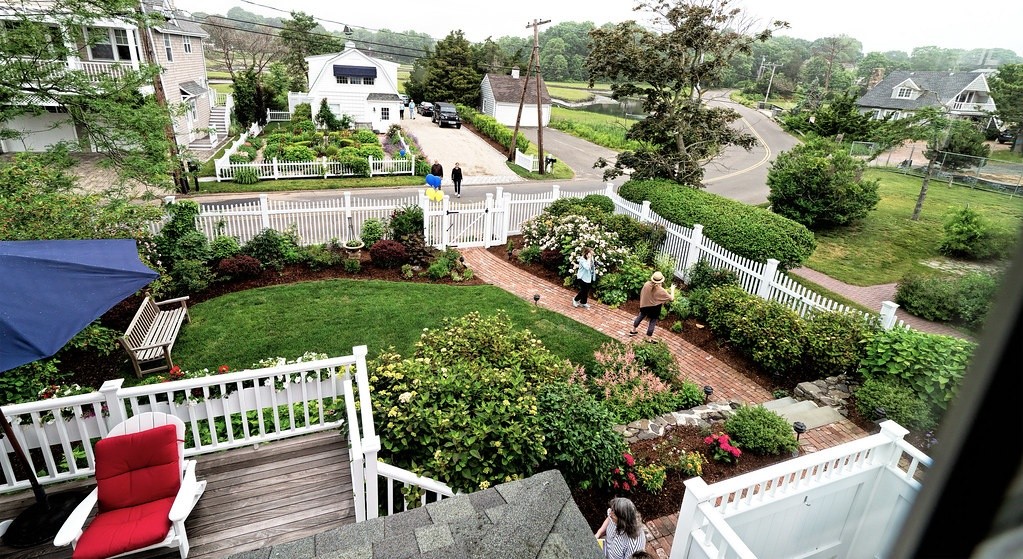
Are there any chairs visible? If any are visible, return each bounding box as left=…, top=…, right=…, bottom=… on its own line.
left=53, top=412, right=207, bottom=559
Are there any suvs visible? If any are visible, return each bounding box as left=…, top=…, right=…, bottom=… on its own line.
left=431, top=101, right=463, bottom=129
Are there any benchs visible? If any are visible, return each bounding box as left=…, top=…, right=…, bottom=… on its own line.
left=118, top=290, right=191, bottom=377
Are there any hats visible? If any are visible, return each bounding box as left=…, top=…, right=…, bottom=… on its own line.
left=651, top=272, right=665, bottom=283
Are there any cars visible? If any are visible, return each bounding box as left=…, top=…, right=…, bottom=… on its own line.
left=400, top=94, right=410, bottom=106
left=417, top=101, right=434, bottom=117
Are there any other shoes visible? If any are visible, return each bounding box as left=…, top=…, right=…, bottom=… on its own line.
left=630, top=331, right=638, bottom=336
left=645, top=339, right=657, bottom=345
left=580, top=304, right=590, bottom=308
left=455, top=192, right=458, bottom=196
left=457, top=194, right=461, bottom=198
left=573, top=297, right=578, bottom=308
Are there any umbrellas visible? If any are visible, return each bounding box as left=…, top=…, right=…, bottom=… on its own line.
left=0, top=238, right=160, bottom=512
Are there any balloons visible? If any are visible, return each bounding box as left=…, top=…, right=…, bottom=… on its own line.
left=426, top=188, right=435, bottom=201
left=435, top=191, right=444, bottom=202
left=400, top=149, right=405, bottom=157
left=434, top=176, right=440, bottom=188
left=426, top=174, right=435, bottom=186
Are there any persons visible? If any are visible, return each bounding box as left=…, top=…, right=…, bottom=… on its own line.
left=627, top=550, right=655, bottom=559
left=408, top=99, right=416, bottom=119
left=430, top=159, right=443, bottom=190
left=400, top=101, right=405, bottom=120
left=593, top=497, right=646, bottom=559
left=572, top=248, right=595, bottom=308
left=627, top=271, right=676, bottom=343
left=451, top=162, right=463, bottom=198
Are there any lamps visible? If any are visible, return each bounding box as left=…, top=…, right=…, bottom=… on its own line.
left=792, top=421, right=806, bottom=434
left=704, top=386, right=713, bottom=395
left=534, top=294, right=541, bottom=301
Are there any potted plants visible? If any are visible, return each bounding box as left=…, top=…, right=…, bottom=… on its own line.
left=344, top=240, right=364, bottom=258
